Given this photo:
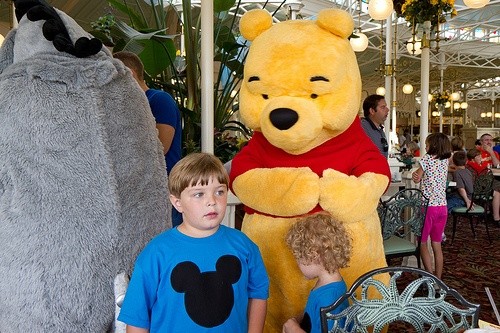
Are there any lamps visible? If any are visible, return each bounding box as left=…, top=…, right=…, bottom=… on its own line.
left=375, top=76, right=468, bottom=116
left=348, top=0, right=367, bottom=52
left=368, top=0, right=394, bottom=20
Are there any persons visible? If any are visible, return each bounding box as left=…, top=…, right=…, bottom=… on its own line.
left=282, top=211, right=360, bottom=333
left=117, top=155, right=270, bottom=333
left=412, top=133, right=452, bottom=280
left=440, top=134, right=500, bottom=241
left=112, top=52, right=183, bottom=228
left=361, top=94, right=390, bottom=160
left=396, top=127, right=420, bottom=172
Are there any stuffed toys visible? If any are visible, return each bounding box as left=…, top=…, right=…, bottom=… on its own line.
left=229, top=9, right=392, bottom=333
left=0, top=1, right=171, bottom=333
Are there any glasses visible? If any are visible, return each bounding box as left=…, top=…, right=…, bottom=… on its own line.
left=381, top=137, right=388, bottom=152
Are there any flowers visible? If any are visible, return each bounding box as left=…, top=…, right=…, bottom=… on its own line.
left=431, top=89, right=449, bottom=107
left=401, top=0, right=457, bottom=23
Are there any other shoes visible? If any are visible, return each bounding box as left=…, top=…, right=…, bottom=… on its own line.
left=494, top=219, right=500, bottom=228
left=423, top=283, right=439, bottom=290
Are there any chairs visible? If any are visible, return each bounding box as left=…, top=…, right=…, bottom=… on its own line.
left=376, top=188, right=429, bottom=294
left=320, top=266, right=481, bottom=333
left=451, top=168, right=494, bottom=243
